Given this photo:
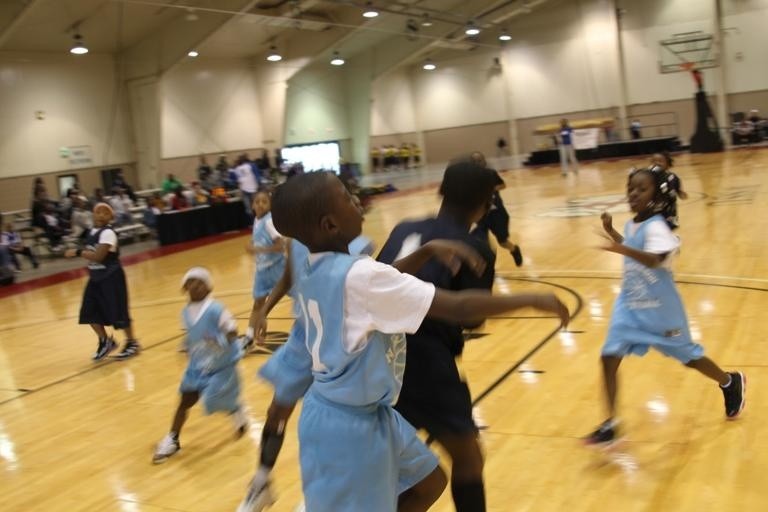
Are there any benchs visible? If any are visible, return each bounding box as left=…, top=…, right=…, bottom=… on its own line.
left=1, top=178, right=255, bottom=253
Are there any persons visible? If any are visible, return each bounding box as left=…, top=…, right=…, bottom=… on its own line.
left=239, top=191, right=294, bottom=356
left=160, top=148, right=303, bottom=211
left=579, top=166, right=748, bottom=446
left=557, top=116, right=582, bottom=176
left=375, top=157, right=505, bottom=512
left=1, top=169, right=164, bottom=270
left=270, top=168, right=570, bottom=512
left=151, top=267, right=247, bottom=465
left=65, top=201, right=144, bottom=364
left=649, top=152, right=688, bottom=228
left=371, top=143, right=421, bottom=168
left=630, top=119, right=643, bottom=140
left=730, top=108, right=767, bottom=144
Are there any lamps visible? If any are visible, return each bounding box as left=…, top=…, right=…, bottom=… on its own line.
left=265, top=45, right=284, bottom=62
left=329, top=50, right=346, bottom=67
left=419, top=13, right=433, bottom=27
left=422, top=57, right=437, bottom=70
left=497, top=28, right=512, bottom=41
left=463, top=20, right=481, bottom=36
left=68, top=21, right=89, bottom=56
left=359, top=2, right=380, bottom=18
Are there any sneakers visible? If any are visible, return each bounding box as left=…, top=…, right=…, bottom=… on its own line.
left=510, top=244, right=522, bottom=266
left=91, top=335, right=120, bottom=362
left=719, top=371, right=746, bottom=418
left=237, top=476, right=278, bottom=512
left=241, top=335, right=256, bottom=355
left=112, top=340, right=139, bottom=359
left=152, top=438, right=181, bottom=464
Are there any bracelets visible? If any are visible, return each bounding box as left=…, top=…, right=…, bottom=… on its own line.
left=76, top=249, right=83, bottom=257
